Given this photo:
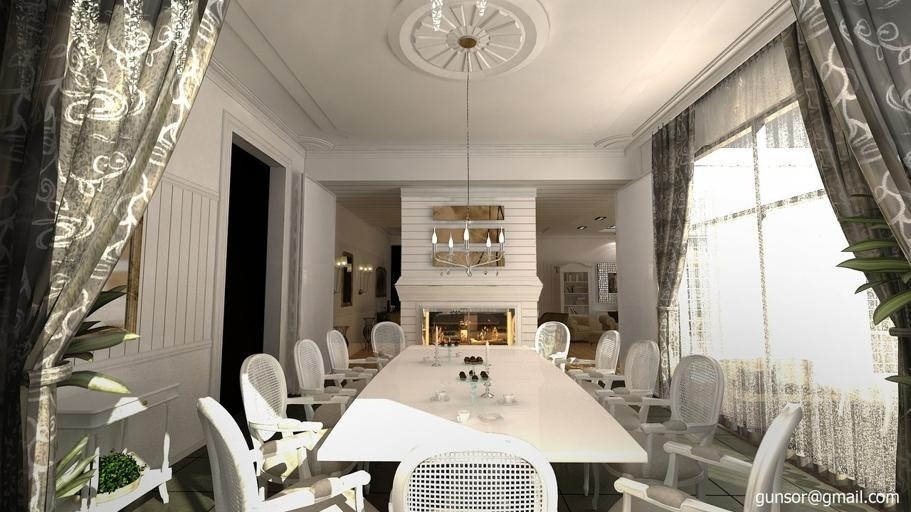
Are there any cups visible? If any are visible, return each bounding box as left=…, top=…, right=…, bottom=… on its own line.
left=503, top=393, right=513, bottom=404
left=436, top=390, right=446, bottom=401
left=457, top=409, right=470, bottom=422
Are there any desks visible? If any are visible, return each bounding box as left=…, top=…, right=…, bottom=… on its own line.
left=54, top=381, right=183, bottom=512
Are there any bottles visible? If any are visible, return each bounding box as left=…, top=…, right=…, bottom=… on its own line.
left=565, top=272, right=579, bottom=282
left=492, top=326, right=499, bottom=340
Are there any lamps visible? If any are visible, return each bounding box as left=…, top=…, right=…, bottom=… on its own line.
left=357, top=264, right=374, bottom=295
left=335, top=255, right=348, bottom=296
left=430, top=37, right=503, bottom=275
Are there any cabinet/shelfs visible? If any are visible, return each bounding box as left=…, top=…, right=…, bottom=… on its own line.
left=559, top=260, right=595, bottom=313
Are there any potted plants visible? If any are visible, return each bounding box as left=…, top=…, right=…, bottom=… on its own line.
left=72, top=448, right=148, bottom=502
